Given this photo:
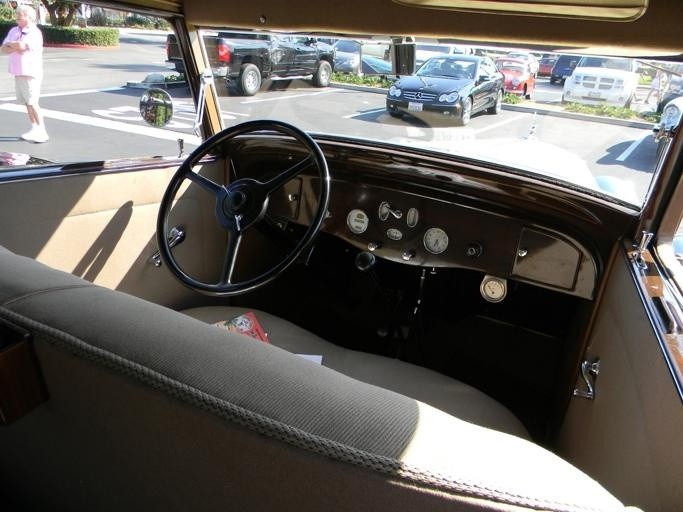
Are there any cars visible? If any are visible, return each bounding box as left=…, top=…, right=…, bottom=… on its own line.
left=336, top=39, right=398, bottom=75
left=494, top=51, right=640, bottom=108
left=385, top=53, right=505, bottom=126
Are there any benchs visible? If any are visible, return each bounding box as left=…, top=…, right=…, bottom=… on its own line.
left=0, top=243, right=644, bottom=512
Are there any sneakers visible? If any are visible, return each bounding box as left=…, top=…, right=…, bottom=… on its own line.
left=21, top=127, right=49, bottom=142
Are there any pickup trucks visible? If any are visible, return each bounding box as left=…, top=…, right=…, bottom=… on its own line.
left=166, top=27, right=335, bottom=97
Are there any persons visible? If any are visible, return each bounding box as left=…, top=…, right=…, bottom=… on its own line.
left=633, top=92, right=643, bottom=102
left=0, top=5, right=50, bottom=143
left=644, top=69, right=662, bottom=104
